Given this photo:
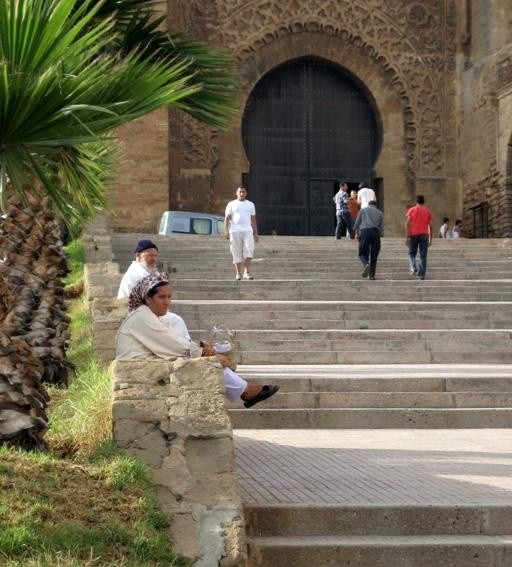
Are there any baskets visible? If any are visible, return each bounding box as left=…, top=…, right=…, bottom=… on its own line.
left=208, top=324, right=237, bottom=372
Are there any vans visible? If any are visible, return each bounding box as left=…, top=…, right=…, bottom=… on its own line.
left=158, top=212, right=230, bottom=238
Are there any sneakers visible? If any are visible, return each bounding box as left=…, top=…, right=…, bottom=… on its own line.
left=361, top=264, right=371, bottom=277
left=242, top=272, right=253, bottom=280
left=236, top=274, right=241, bottom=280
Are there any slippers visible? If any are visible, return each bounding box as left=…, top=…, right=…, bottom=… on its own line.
left=243, top=384, right=280, bottom=409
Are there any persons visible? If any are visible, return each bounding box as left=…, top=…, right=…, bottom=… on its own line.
left=354, top=200, right=383, bottom=279
left=451, top=218, right=466, bottom=238
left=224, top=185, right=259, bottom=280
left=114, top=270, right=280, bottom=408
left=348, top=189, right=360, bottom=239
left=333, top=181, right=355, bottom=239
left=356, top=181, right=377, bottom=207
left=116, top=239, right=167, bottom=298
left=403, top=194, right=434, bottom=280
left=438, top=216, right=450, bottom=238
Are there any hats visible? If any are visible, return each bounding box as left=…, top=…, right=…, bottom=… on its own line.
left=134, top=240, right=158, bottom=254
